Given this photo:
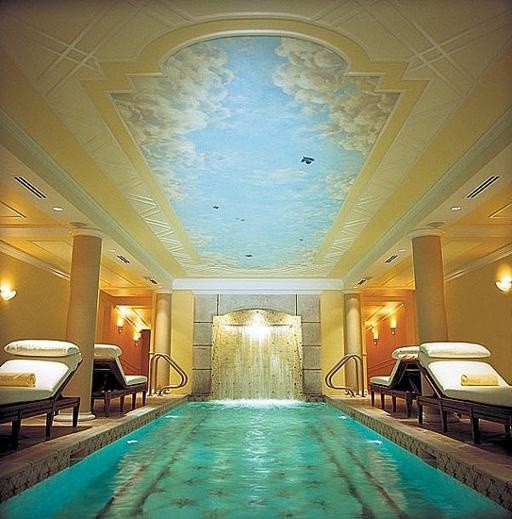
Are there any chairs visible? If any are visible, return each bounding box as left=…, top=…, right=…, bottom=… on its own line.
left=1, top=339, right=82, bottom=451
left=365, top=344, right=419, bottom=419
left=93, top=343, right=149, bottom=418
left=413, top=341, right=511, bottom=448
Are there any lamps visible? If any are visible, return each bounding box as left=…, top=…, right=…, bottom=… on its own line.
left=495, top=280, right=511, bottom=293
left=117, top=319, right=141, bottom=347
left=372, top=321, right=396, bottom=345
left=1, top=289, right=18, bottom=301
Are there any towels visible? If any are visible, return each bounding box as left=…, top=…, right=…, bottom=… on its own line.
left=458, top=373, right=499, bottom=386
left=1, top=371, right=37, bottom=387
